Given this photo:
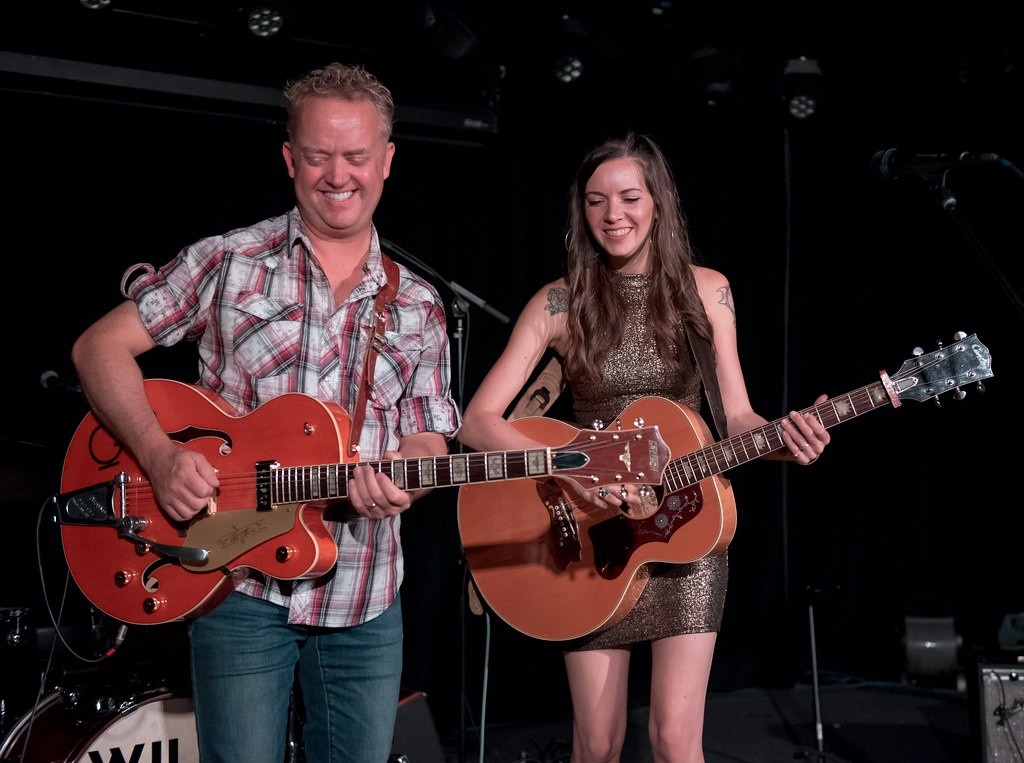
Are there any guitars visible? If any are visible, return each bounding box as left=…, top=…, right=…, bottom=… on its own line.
left=458, top=330, right=994, bottom=642
left=59, top=378, right=671, bottom=627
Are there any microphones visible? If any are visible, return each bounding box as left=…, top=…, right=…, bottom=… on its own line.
left=870, top=147, right=998, bottom=181
left=40, top=370, right=60, bottom=388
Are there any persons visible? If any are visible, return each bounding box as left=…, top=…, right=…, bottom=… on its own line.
left=455, top=131, right=831, bottom=763
left=72, top=63, right=464, bottom=763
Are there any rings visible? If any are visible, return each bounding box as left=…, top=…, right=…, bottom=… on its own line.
left=794, top=450, right=802, bottom=458
left=598, top=487, right=609, bottom=498
left=801, top=442, right=810, bottom=451
left=367, top=502, right=377, bottom=510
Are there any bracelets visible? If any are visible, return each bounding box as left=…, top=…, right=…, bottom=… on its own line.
left=796, top=454, right=821, bottom=465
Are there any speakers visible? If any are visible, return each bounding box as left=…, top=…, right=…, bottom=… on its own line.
left=386, top=690, right=445, bottom=763
left=967, top=663, right=1024, bottom=763
